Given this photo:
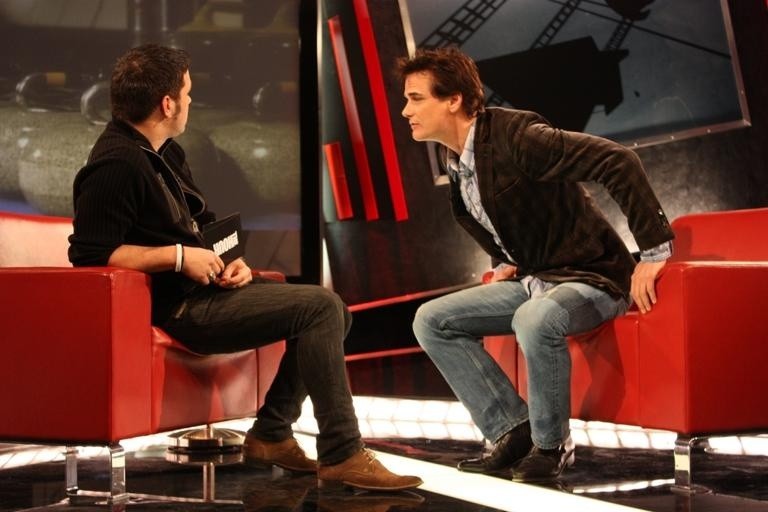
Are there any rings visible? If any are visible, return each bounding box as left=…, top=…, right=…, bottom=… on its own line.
left=208, top=271, right=216, bottom=282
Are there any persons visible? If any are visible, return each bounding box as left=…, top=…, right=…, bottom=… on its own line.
left=238, top=470, right=425, bottom=512
left=67, top=43, right=424, bottom=492
left=392, top=46, right=675, bottom=483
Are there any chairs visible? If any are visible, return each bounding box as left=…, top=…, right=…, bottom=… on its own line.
left=0, top=211, right=289, bottom=506
left=483, top=206, right=768, bottom=496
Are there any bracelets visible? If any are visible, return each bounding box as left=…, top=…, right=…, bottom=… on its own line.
left=174, top=243, right=183, bottom=273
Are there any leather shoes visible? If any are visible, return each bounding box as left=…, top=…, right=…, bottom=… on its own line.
left=458, top=423, right=530, bottom=471
left=512, top=477, right=575, bottom=493
left=314, top=491, right=426, bottom=512
left=318, top=448, right=423, bottom=490
left=241, top=475, right=317, bottom=511
left=513, top=446, right=575, bottom=478
left=244, top=432, right=317, bottom=473
